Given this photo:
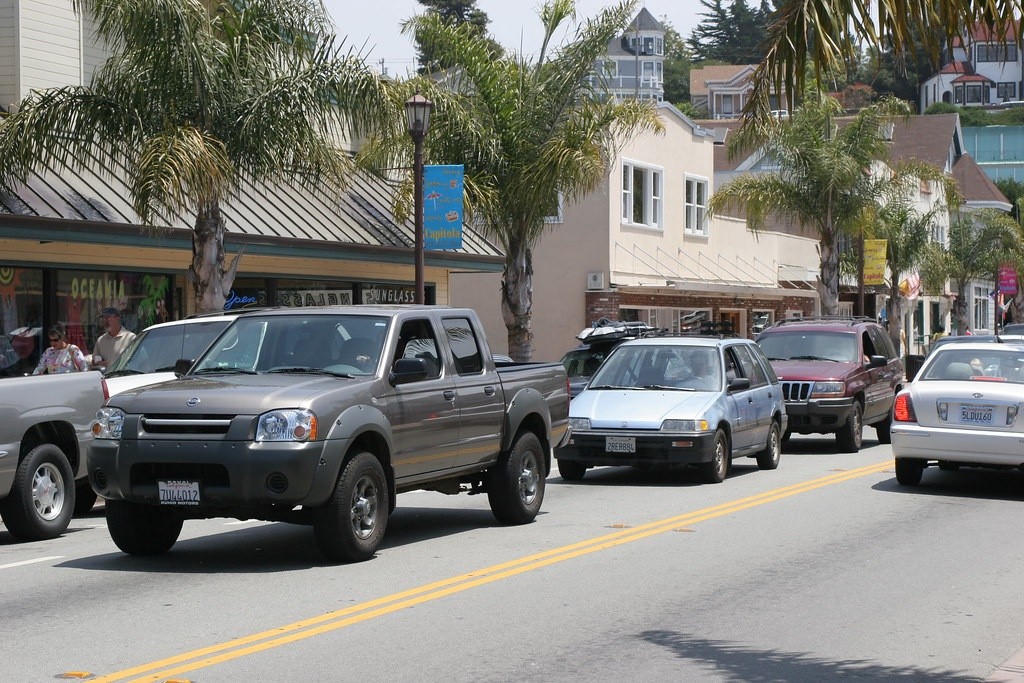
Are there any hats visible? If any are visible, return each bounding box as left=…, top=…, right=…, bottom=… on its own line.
left=99, top=307, right=122, bottom=317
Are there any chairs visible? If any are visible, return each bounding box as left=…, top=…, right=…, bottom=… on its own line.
left=342, top=338, right=378, bottom=366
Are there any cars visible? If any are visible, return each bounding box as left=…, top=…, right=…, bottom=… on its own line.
left=553, top=333, right=790, bottom=483
left=923, top=321, right=1023, bottom=372
left=890, top=342, right=1024, bottom=486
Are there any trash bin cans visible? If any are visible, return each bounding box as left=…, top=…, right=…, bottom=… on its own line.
left=905, top=354, right=924, bottom=381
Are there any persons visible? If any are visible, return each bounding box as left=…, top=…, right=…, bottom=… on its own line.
left=356, top=329, right=392, bottom=373
left=32, top=307, right=149, bottom=375
left=964, top=326, right=972, bottom=335
left=932, top=326, right=947, bottom=341
left=682, top=352, right=711, bottom=381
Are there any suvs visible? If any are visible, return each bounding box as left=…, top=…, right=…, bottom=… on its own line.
left=72, top=306, right=355, bottom=517
left=749, top=315, right=906, bottom=455
left=558, top=319, right=695, bottom=404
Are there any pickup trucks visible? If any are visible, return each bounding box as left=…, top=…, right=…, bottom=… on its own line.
left=84, top=307, right=570, bottom=563
left=0, top=370, right=112, bottom=542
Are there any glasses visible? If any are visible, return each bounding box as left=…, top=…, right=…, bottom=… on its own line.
left=49, top=338, right=61, bottom=342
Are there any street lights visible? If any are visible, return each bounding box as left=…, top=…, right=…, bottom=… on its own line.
left=401, top=90, right=434, bottom=304
